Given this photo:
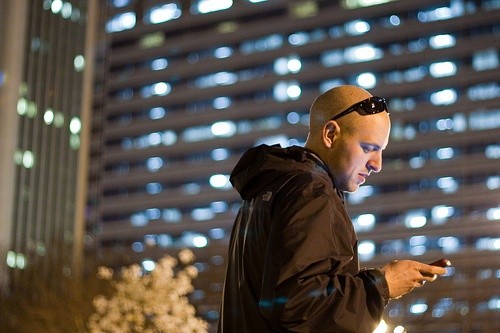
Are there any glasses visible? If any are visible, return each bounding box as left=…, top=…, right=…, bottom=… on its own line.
left=331, top=91, right=395, bottom=121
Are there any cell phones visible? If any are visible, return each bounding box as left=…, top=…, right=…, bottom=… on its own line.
left=430, top=257, right=452, bottom=267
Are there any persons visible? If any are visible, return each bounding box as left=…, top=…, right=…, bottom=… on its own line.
left=217, top=85, right=447, bottom=333
